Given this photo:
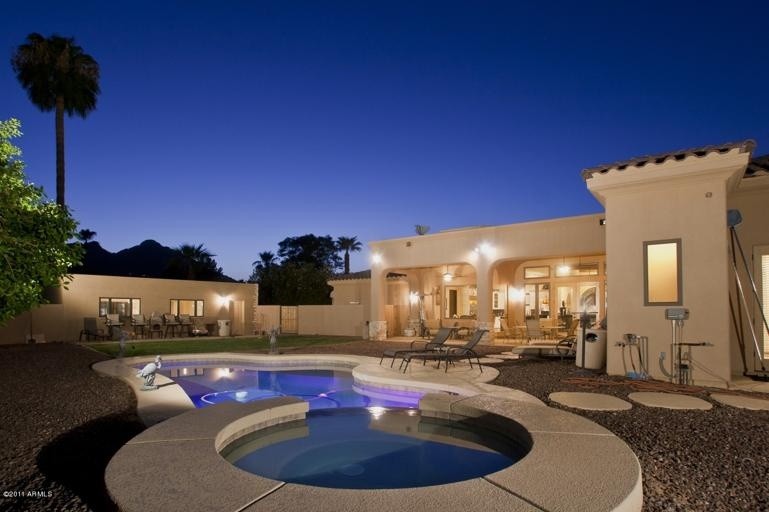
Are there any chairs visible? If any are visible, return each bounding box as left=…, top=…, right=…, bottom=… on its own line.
left=378, top=318, right=490, bottom=375
left=77, top=311, right=210, bottom=343
left=498, top=314, right=565, bottom=344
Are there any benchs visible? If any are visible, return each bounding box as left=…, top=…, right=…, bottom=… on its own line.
left=511, top=334, right=576, bottom=363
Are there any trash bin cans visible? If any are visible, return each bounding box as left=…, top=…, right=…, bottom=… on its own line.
left=575, top=328, right=606, bottom=370
left=217, top=320, right=231, bottom=336
